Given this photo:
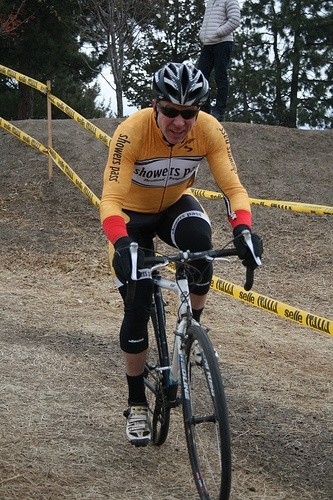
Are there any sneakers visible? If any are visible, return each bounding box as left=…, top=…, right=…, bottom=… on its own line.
left=191, top=332, right=219, bottom=366
left=124, top=399, right=152, bottom=447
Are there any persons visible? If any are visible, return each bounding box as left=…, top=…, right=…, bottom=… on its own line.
left=99, top=62, right=264, bottom=446
left=197, top=0, right=241, bottom=122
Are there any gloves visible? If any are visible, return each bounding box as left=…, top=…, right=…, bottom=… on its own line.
left=232, top=224, right=263, bottom=269
left=111, top=235, right=144, bottom=286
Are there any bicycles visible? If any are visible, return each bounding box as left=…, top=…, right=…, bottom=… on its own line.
left=122, top=230, right=264, bottom=500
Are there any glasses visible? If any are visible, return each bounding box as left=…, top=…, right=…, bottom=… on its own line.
left=155, top=101, right=200, bottom=120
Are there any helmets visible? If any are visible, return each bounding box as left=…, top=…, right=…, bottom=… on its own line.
left=150, top=62, right=208, bottom=108
